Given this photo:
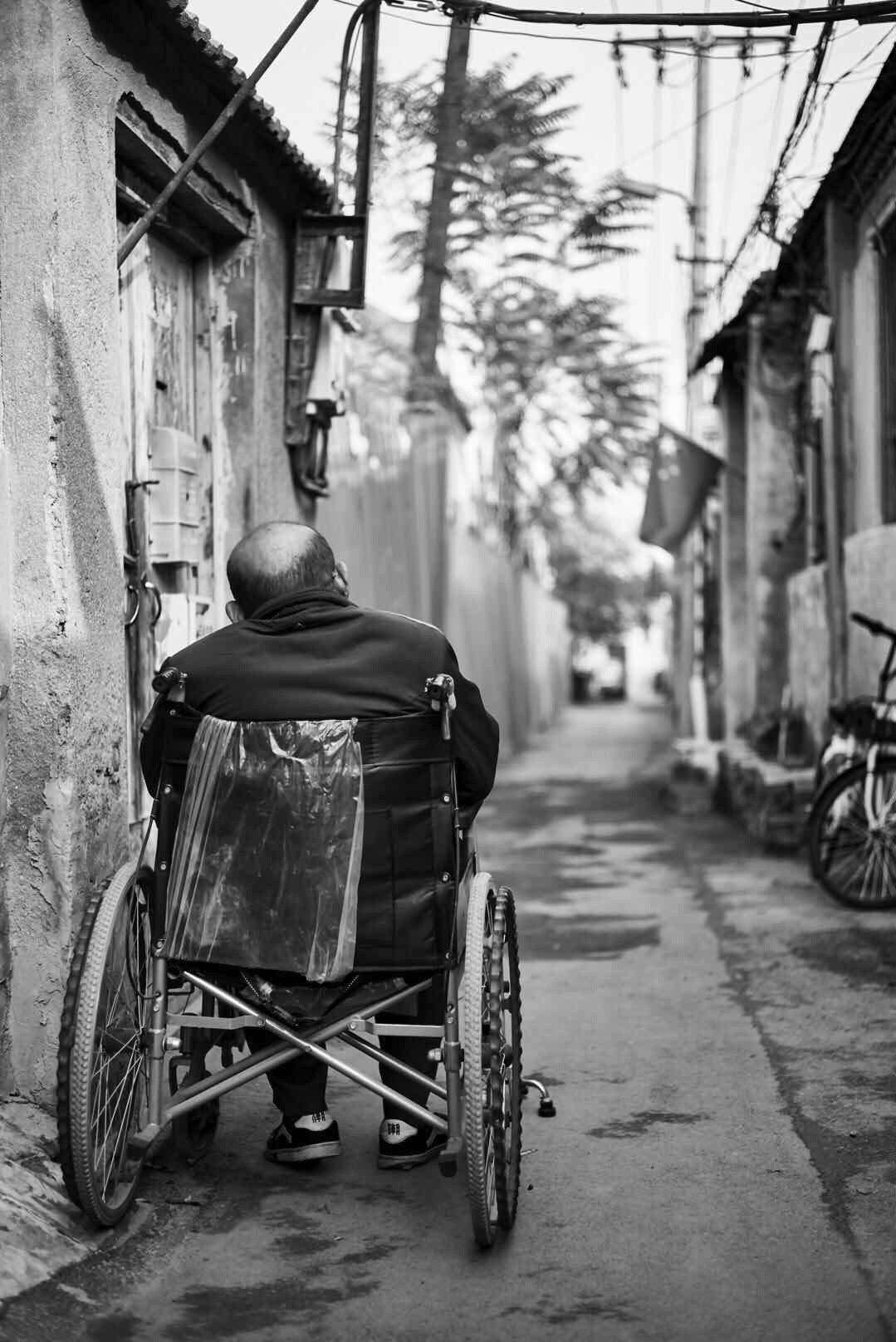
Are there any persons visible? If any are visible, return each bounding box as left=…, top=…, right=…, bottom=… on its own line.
left=141, top=519, right=502, bottom=1169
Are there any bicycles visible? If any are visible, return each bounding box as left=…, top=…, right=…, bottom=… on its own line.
left=807, top=612, right=896, bottom=912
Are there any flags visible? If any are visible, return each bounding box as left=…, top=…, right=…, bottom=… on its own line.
left=638, top=423, right=725, bottom=555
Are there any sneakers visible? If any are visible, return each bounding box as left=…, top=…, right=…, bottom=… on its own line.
left=376, top=1110, right=448, bottom=1171
left=264, top=1113, right=341, bottom=1163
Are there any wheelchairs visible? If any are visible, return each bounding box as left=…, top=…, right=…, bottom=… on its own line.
left=53, top=667, right=557, bottom=1255
